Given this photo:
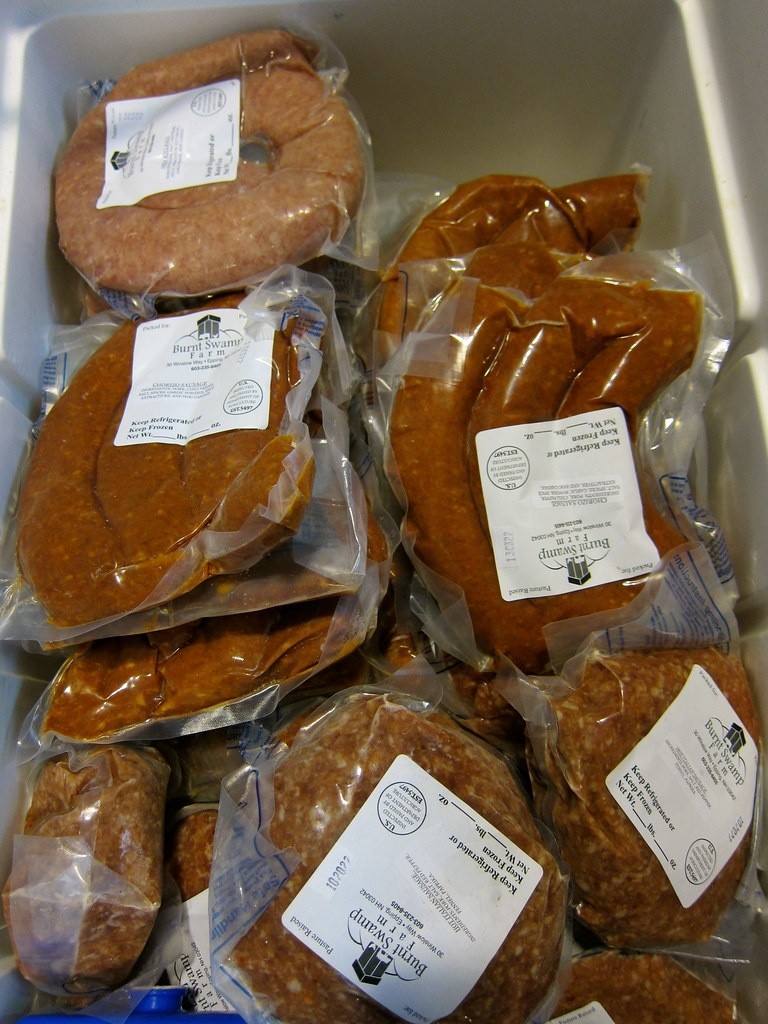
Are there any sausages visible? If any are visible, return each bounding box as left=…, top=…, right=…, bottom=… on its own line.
left=9, top=30, right=707, bottom=729
